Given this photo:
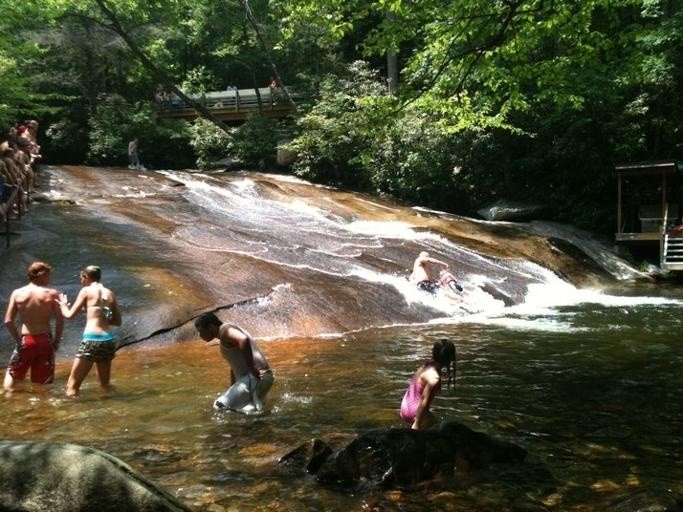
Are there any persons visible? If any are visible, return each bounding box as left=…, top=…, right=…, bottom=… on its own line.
left=2, top=261, right=62, bottom=388
left=0, top=119, right=42, bottom=220
left=396, top=337, right=458, bottom=429
left=193, top=312, right=272, bottom=413
left=50, top=266, right=121, bottom=397
